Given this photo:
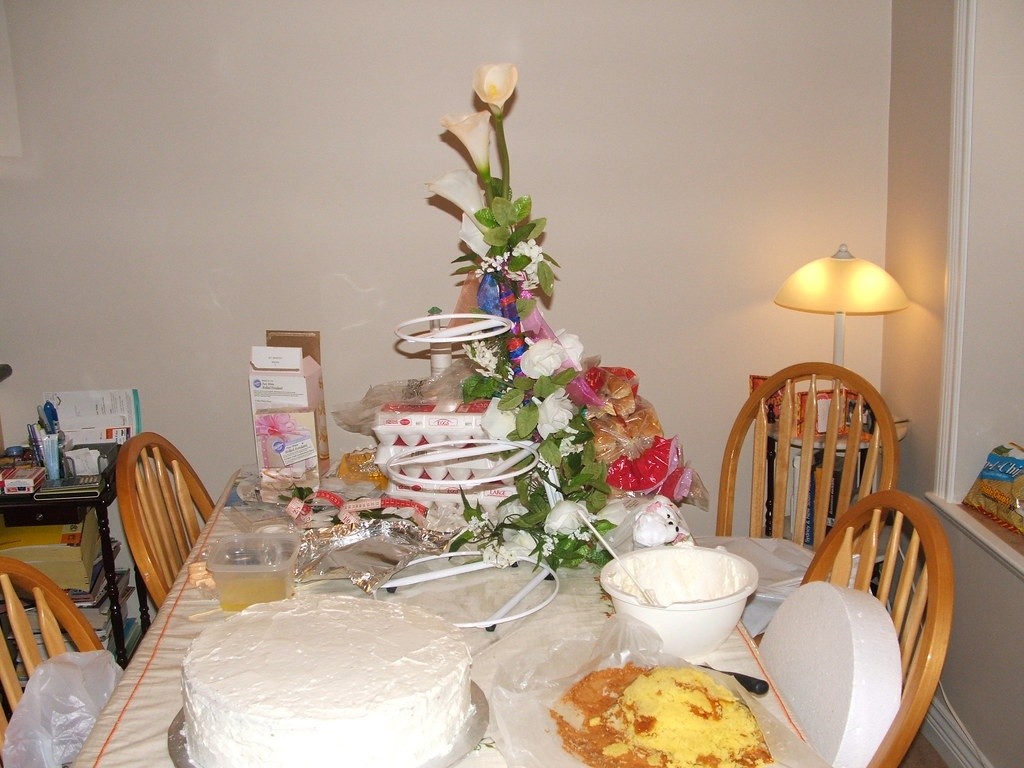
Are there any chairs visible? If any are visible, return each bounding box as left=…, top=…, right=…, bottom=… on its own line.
left=0, top=555, right=125, bottom=768
left=714, top=361, right=898, bottom=553
left=755, top=489, right=954, bottom=768
left=116, top=431, right=216, bottom=611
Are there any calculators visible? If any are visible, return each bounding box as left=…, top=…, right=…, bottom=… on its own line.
left=40, top=474, right=103, bottom=492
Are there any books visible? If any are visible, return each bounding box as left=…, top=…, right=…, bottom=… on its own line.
left=804, top=454, right=857, bottom=545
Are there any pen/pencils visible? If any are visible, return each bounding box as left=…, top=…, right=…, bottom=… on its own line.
left=27, top=406, right=65, bottom=480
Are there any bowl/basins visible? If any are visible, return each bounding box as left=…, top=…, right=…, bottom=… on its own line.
left=601, top=546, right=760, bottom=655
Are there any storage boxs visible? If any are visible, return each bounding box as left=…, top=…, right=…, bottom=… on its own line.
left=247, top=326, right=330, bottom=483
left=0, top=467, right=47, bottom=494
left=0, top=514, right=98, bottom=592
left=202, top=531, right=302, bottom=611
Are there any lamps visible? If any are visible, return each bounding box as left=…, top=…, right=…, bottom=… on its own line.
left=774, top=243, right=909, bottom=390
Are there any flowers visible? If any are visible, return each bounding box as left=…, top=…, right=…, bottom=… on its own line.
left=426, top=62, right=613, bottom=563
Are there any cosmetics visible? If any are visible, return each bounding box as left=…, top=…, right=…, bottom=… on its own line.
left=43, top=434, right=60, bottom=480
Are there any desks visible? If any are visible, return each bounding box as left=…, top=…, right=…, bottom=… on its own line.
left=65, top=466, right=821, bottom=768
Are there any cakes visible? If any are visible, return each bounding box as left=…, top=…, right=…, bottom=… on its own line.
left=178, top=593, right=473, bottom=768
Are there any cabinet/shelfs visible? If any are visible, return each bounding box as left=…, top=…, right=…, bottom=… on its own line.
left=0, top=443, right=151, bottom=666
left=767, top=415, right=910, bottom=565
